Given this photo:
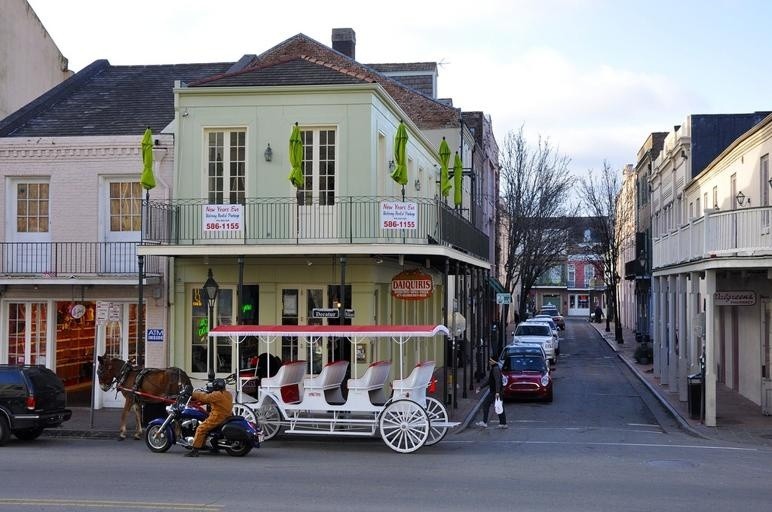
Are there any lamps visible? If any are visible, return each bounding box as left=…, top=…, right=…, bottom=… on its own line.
left=415, top=180, right=421, bottom=192
left=735, top=188, right=752, bottom=208
left=388, top=160, right=395, bottom=170
left=264, top=143, right=274, bottom=164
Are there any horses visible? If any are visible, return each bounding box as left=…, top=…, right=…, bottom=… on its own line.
left=96, top=352, right=192, bottom=441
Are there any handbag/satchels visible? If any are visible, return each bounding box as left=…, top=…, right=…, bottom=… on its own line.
left=495, top=400, right=504, bottom=414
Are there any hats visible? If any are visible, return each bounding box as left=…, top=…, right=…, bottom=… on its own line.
left=490, top=356, right=498, bottom=363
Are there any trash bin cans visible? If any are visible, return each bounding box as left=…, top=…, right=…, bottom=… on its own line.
left=687, top=372, right=702, bottom=420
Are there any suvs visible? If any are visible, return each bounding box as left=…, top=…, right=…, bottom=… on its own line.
left=491, top=342, right=556, bottom=405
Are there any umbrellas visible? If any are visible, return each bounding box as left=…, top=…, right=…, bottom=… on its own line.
left=438, top=137, right=452, bottom=204
left=288, top=122, right=304, bottom=197
left=390, top=119, right=408, bottom=202
left=141, top=127, right=156, bottom=235
left=453, top=151, right=463, bottom=212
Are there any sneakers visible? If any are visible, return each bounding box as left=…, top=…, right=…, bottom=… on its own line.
left=497, top=425, right=507, bottom=429
left=475, top=421, right=487, bottom=427
left=185, top=451, right=199, bottom=457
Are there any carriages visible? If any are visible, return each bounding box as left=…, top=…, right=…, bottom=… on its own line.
left=91, top=316, right=468, bottom=455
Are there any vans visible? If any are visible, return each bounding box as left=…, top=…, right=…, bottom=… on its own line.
left=0, top=362, right=74, bottom=445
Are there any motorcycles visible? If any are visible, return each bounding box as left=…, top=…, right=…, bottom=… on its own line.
left=143, top=383, right=261, bottom=458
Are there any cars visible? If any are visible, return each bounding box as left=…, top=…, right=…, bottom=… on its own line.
left=517, top=301, right=568, bottom=362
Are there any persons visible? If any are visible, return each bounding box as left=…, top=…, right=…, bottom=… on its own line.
left=475, top=356, right=508, bottom=429
left=595, top=305, right=605, bottom=321
left=184, top=379, right=232, bottom=457
left=514, top=311, right=519, bottom=328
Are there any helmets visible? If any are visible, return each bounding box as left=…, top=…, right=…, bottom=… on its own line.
left=213, top=378, right=225, bottom=390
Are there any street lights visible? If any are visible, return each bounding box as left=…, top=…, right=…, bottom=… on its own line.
left=614, top=271, right=625, bottom=345
left=200, top=267, right=220, bottom=409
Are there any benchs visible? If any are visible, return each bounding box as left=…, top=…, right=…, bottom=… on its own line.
left=301, top=360, right=349, bottom=390
left=347, top=359, right=390, bottom=389
left=259, top=357, right=307, bottom=389
left=393, top=357, right=436, bottom=389
